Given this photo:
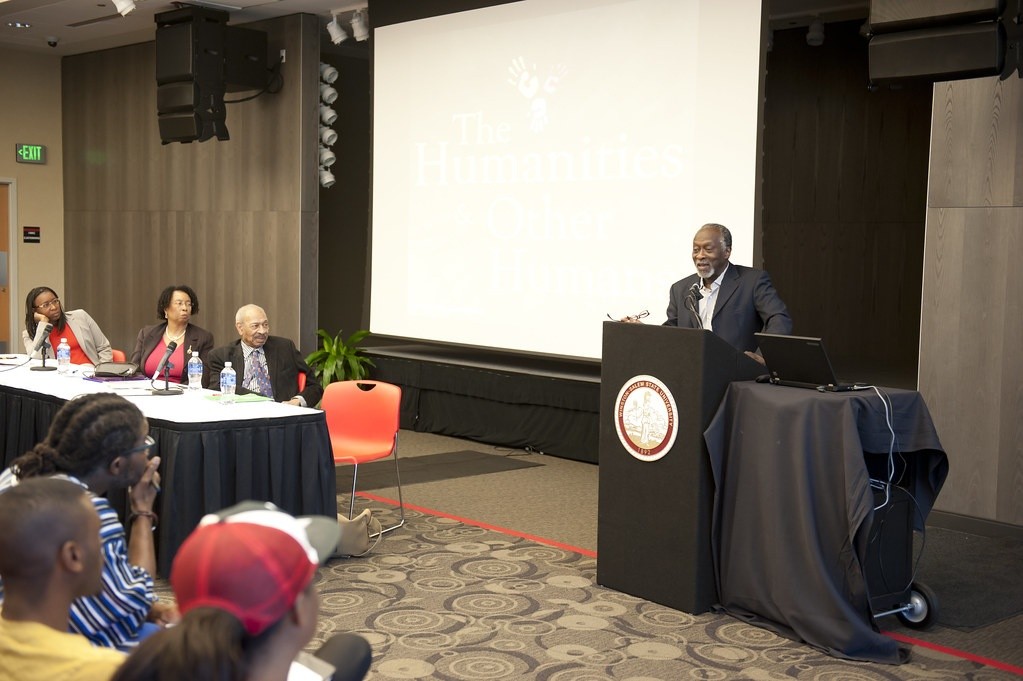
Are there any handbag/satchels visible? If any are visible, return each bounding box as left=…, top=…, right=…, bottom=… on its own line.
left=337, top=508, right=382, bottom=557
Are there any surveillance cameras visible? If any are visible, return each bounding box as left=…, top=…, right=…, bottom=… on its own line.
left=46, top=36, right=59, bottom=46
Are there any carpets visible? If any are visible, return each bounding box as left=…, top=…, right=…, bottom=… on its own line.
left=913, top=526, right=1023, bottom=634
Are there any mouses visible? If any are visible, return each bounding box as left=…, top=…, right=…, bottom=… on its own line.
left=755, top=374, right=771, bottom=382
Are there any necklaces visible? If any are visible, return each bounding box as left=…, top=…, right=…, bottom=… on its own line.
left=166, top=325, right=186, bottom=341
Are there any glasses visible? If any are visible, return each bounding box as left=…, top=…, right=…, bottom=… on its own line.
left=33, top=299, right=60, bottom=309
left=607, top=309, right=650, bottom=323
left=126, top=436, right=156, bottom=454
left=170, top=300, right=195, bottom=308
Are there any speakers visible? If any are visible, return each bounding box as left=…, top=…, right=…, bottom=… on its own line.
left=867, top=0, right=1007, bottom=88
left=155, top=18, right=224, bottom=143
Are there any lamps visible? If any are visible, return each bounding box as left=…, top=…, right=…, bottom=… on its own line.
left=319, top=121, right=338, bottom=146
left=321, top=63, right=338, bottom=84
left=319, top=143, right=336, bottom=167
left=349, top=9, right=370, bottom=41
left=112, top=0, right=137, bottom=17
left=326, top=10, right=349, bottom=47
left=320, top=81, right=339, bottom=106
left=319, top=164, right=336, bottom=188
left=320, top=102, right=338, bottom=125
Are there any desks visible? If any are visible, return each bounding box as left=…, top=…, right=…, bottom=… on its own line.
left=1, top=354, right=338, bottom=577
left=703, top=381, right=952, bottom=667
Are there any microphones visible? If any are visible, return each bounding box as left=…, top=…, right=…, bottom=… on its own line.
left=30, top=324, right=53, bottom=359
left=151, top=341, right=177, bottom=381
left=690, top=282, right=700, bottom=292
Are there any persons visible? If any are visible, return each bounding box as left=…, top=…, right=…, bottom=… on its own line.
left=130, top=285, right=215, bottom=389
left=207, top=304, right=324, bottom=408
left=0, top=392, right=173, bottom=654
left=0, top=477, right=130, bottom=681
left=21, top=286, right=113, bottom=366
left=621, top=223, right=793, bottom=367
left=107, top=498, right=344, bottom=681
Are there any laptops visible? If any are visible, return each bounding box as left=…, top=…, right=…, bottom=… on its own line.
left=755, top=332, right=869, bottom=389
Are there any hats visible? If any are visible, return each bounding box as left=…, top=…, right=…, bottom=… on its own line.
left=169, top=501, right=343, bottom=640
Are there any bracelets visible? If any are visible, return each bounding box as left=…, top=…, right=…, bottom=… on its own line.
left=129, top=510, right=159, bottom=531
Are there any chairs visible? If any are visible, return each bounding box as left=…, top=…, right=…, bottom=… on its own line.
left=321, top=379, right=406, bottom=537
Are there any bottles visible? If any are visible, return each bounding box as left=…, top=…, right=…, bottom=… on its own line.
left=188, top=352, right=203, bottom=396
left=57, top=339, right=70, bottom=375
left=220, top=362, right=236, bottom=405
